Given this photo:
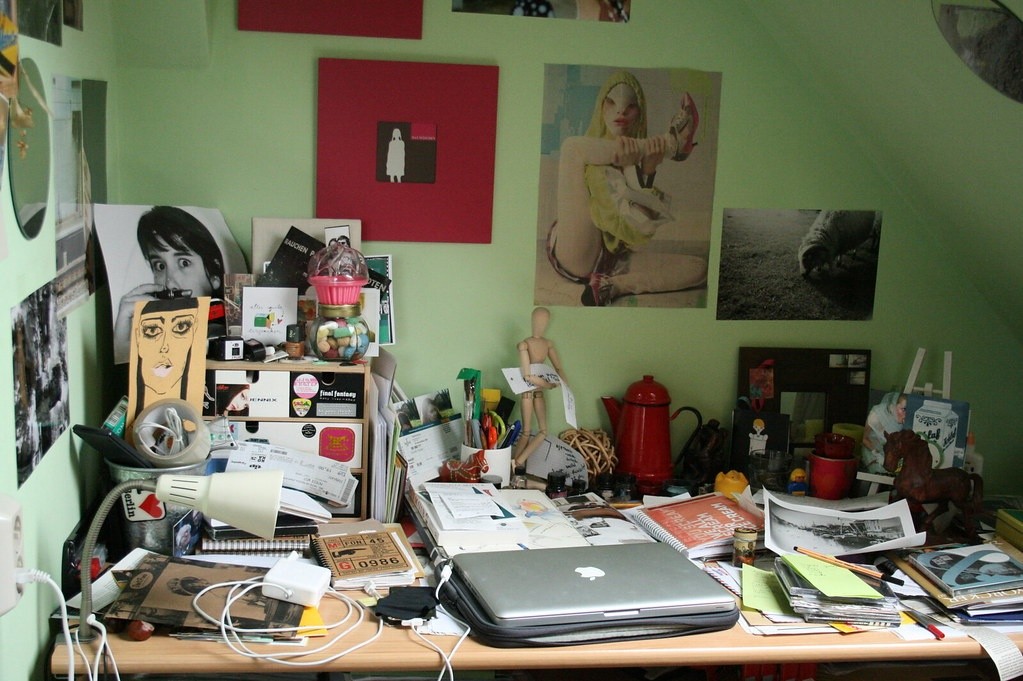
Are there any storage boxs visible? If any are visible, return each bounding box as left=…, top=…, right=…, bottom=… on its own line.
left=211, top=369, right=363, bottom=516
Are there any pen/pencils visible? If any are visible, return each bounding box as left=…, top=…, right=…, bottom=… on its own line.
left=498, top=420, right=522, bottom=449
left=470, top=416, right=488, bottom=449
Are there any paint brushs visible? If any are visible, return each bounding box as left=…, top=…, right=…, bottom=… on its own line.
left=463, top=377, right=476, bottom=446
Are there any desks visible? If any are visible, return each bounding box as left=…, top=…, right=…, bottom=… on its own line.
left=51, top=494, right=1022, bottom=681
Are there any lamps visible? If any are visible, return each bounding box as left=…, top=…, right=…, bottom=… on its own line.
left=76, top=469, right=284, bottom=645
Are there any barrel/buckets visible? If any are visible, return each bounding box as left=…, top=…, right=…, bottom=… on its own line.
left=104, top=458, right=210, bottom=556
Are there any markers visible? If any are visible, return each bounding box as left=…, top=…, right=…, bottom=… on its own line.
left=793, top=546, right=905, bottom=586
left=905, top=610, right=945, bottom=639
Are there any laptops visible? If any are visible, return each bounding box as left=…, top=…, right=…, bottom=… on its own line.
left=453, top=541, right=736, bottom=628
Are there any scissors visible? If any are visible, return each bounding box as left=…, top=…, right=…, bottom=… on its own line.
left=483, top=411, right=506, bottom=449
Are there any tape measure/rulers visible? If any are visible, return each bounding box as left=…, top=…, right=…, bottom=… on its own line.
left=480, top=388, right=516, bottom=424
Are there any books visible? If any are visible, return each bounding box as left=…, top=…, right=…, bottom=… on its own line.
left=195, top=512, right=417, bottom=590
left=408, top=482, right=529, bottom=546
left=633, top=496, right=1023, bottom=627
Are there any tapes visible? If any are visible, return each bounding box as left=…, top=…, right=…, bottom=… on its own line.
left=132, top=398, right=212, bottom=468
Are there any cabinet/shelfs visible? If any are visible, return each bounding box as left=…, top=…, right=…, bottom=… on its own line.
left=203, top=362, right=370, bottom=525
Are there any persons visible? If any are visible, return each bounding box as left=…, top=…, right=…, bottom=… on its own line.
left=216, top=384, right=249, bottom=416
left=955, top=561, right=1022, bottom=585
left=511, top=307, right=567, bottom=474
left=114, top=206, right=225, bottom=364
left=175, top=510, right=203, bottom=557
left=861, top=391, right=907, bottom=475
left=547, top=70, right=708, bottom=306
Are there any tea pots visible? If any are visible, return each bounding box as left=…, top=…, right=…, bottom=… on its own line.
left=601, top=375, right=702, bottom=495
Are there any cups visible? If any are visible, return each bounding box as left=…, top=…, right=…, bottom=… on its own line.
left=808, top=451, right=858, bottom=499
left=832, top=424, right=863, bottom=448
left=814, top=433, right=855, bottom=458
left=461, top=440, right=512, bottom=488
left=748, top=449, right=792, bottom=494
